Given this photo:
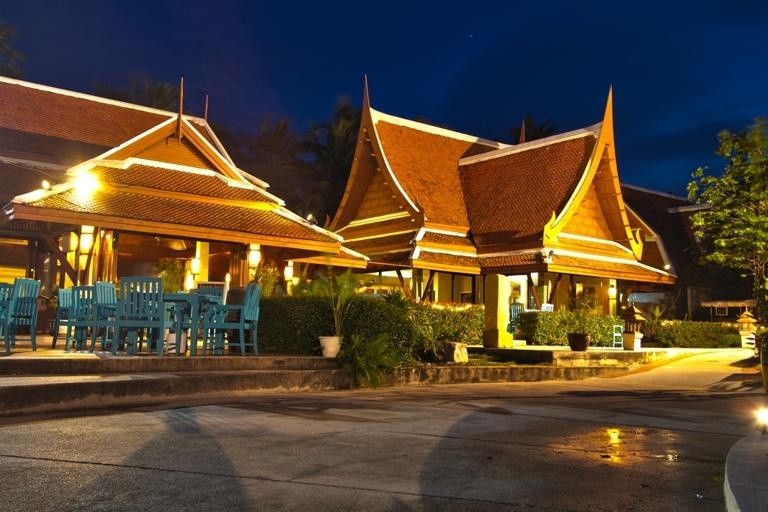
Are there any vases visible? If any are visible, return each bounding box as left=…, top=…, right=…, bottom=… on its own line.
left=318, top=335, right=342, bottom=358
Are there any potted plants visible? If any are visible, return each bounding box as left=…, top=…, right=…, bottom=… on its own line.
left=566, top=292, right=598, bottom=351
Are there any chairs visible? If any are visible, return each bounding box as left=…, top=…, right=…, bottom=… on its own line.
left=1, top=275, right=262, bottom=357
left=612, top=325, right=624, bottom=348
